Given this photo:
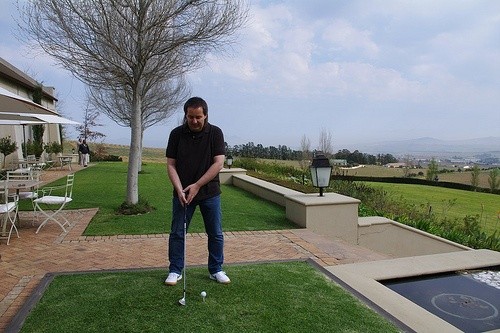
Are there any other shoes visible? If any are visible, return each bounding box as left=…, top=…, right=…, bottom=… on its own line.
left=84, top=165, right=87, bottom=167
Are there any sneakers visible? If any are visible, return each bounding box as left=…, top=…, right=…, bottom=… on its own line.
left=165, top=272, right=182, bottom=285
left=210, top=270, right=230, bottom=284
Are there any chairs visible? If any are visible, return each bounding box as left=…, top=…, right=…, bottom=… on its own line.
left=7, top=154, right=73, bottom=174
left=0, top=193, right=20, bottom=246
left=7, top=171, right=39, bottom=226
left=31, top=173, right=74, bottom=232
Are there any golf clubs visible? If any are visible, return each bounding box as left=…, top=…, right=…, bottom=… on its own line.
left=177, top=192, right=186, bottom=306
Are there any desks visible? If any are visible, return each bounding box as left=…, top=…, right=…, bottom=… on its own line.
left=0, top=180, right=44, bottom=229
left=40, top=160, right=58, bottom=171
left=58, top=156, right=73, bottom=171
left=10, top=160, right=37, bottom=168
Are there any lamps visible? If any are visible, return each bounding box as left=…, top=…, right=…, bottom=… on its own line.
left=311, top=154, right=333, bottom=195
left=226, top=154, right=233, bottom=169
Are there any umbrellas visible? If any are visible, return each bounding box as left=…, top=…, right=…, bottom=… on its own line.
left=0, top=87, right=80, bottom=168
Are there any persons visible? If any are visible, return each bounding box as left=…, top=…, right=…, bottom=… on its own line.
left=77, top=138, right=90, bottom=167
left=165, top=98, right=231, bottom=285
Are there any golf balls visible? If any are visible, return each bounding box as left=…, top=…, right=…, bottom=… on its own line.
left=201, top=291, right=207, bottom=298
